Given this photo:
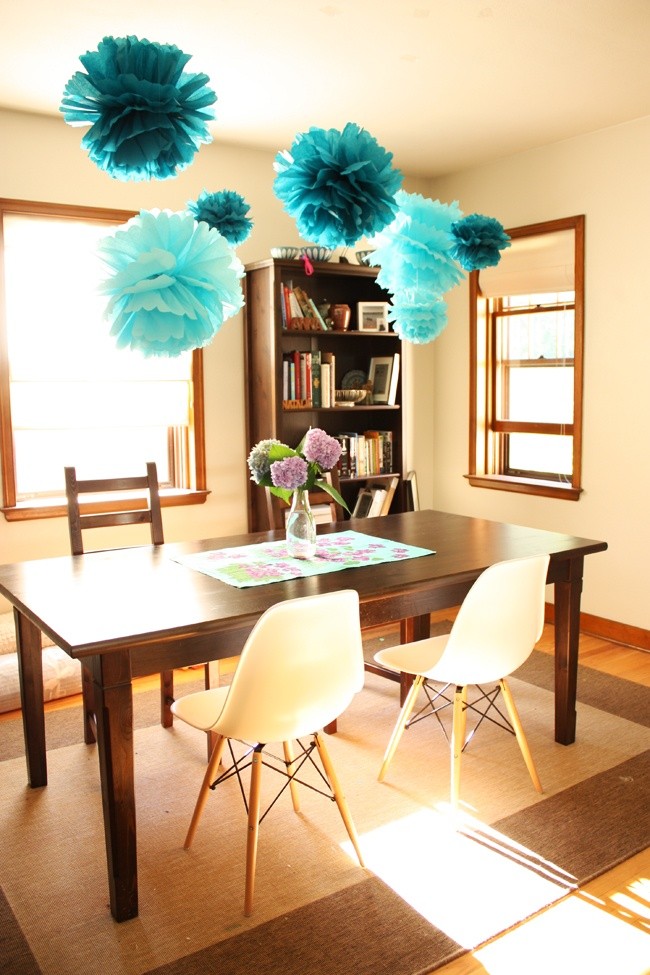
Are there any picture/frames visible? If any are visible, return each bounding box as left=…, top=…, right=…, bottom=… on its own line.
left=407, top=471, right=420, bottom=511
left=351, top=477, right=400, bottom=518
left=357, top=302, right=388, bottom=333
left=368, top=353, right=400, bottom=406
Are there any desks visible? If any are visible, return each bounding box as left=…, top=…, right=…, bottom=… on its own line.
left=0, top=509, right=608, bottom=923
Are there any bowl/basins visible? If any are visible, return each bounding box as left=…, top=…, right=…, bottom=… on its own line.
left=356, top=249, right=375, bottom=266
left=300, top=246, right=332, bottom=262
left=270, top=247, right=299, bottom=259
left=335, top=389, right=367, bottom=406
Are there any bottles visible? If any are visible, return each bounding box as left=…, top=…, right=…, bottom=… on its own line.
left=330, top=303, right=351, bottom=332
left=318, top=304, right=330, bottom=320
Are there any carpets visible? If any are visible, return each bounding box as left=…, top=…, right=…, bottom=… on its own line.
left=0, top=619, right=650, bottom=975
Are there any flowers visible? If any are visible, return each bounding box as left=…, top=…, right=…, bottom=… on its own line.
left=244, top=425, right=349, bottom=514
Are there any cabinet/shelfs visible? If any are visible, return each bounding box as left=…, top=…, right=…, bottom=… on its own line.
left=240, top=258, right=402, bottom=533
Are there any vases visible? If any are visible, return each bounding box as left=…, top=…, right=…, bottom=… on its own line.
left=283, top=487, right=318, bottom=559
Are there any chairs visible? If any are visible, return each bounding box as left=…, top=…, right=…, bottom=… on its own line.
left=170, top=590, right=366, bottom=917
left=373, top=554, right=550, bottom=810
left=265, top=467, right=414, bottom=734
left=65, top=462, right=222, bottom=764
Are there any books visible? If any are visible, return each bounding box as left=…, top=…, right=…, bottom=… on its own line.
left=281, top=350, right=335, bottom=408
left=348, top=478, right=399, bottom=519
left=330, top=430, right=393, bottom=478
left=280, top=280, right=328, bottom=331
